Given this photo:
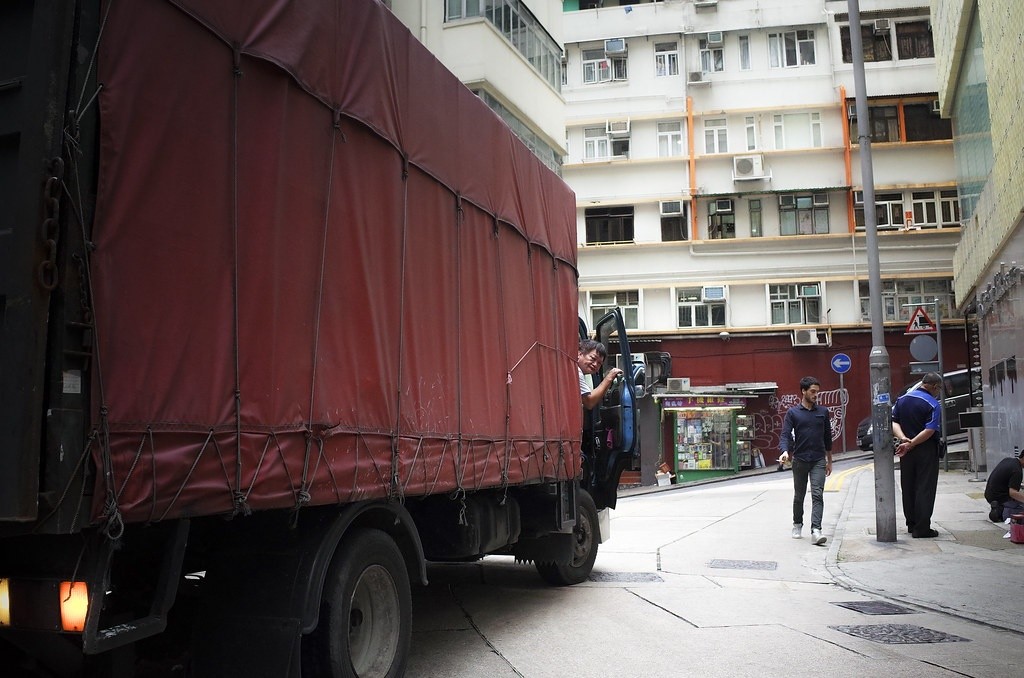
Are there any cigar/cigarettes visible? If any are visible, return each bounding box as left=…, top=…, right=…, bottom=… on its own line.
left=776, top=459, right=784, bottom=463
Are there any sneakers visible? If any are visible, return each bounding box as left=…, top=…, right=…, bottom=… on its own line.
left=812, top=529, right=827, bottom=545
left=792, top=524, right=803, bottom=538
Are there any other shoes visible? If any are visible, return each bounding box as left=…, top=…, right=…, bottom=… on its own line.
left=916, top=529, right=938, bottom=538
left=989, top=501, right=1000, bottom=522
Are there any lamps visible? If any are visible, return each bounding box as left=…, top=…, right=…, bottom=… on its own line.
left=800, top=285, right=818, bottom=295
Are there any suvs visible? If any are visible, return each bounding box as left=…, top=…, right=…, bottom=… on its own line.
left=856, top=366, right=982, bottom=457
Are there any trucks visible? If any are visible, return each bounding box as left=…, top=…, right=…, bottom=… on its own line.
left=0, top=0, right=670, bottom=678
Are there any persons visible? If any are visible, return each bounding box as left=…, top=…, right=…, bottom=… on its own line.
left=577, top=338, right=622, bottom=411
left=689, top=452, right=698, bottom=470
left=984, top=448, right=1024, bottom=523
left=891, top=371, right=943, bottom=538
left=776, top=376, right=833, bottom=546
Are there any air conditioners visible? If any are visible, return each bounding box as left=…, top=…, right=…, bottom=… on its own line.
left=813, top=192, right=829, bottom=207
left=707, top=31, right=722, bottom=47
left=701, top=286, right=726, bottom=302
left=693, top=0, right=719, bottom=7
left=660, top=201, right=684, bottom=219
left=779, top=194, right=796, bottom=209
left=873, top=18, right=892, bottom=36
left=846, top=105, right=858, bottom=120
left=616, top=353, right=647, bottom=372
left=792, top=328, right=819, bottom=347
left=854, top=191, right=863, bottom=207
left=716, top=199, right=733, bottom=212
left=687, top=70, right=711, bottom=83
left=732, top=154, right=764, bottom=179
left=604, top=39, right=627, bottom=55
left=666, top=377, right=690, bottom=394
left=610, top=121, right=628, bottom=134
left=933, top=98, right=941, bottom=112
